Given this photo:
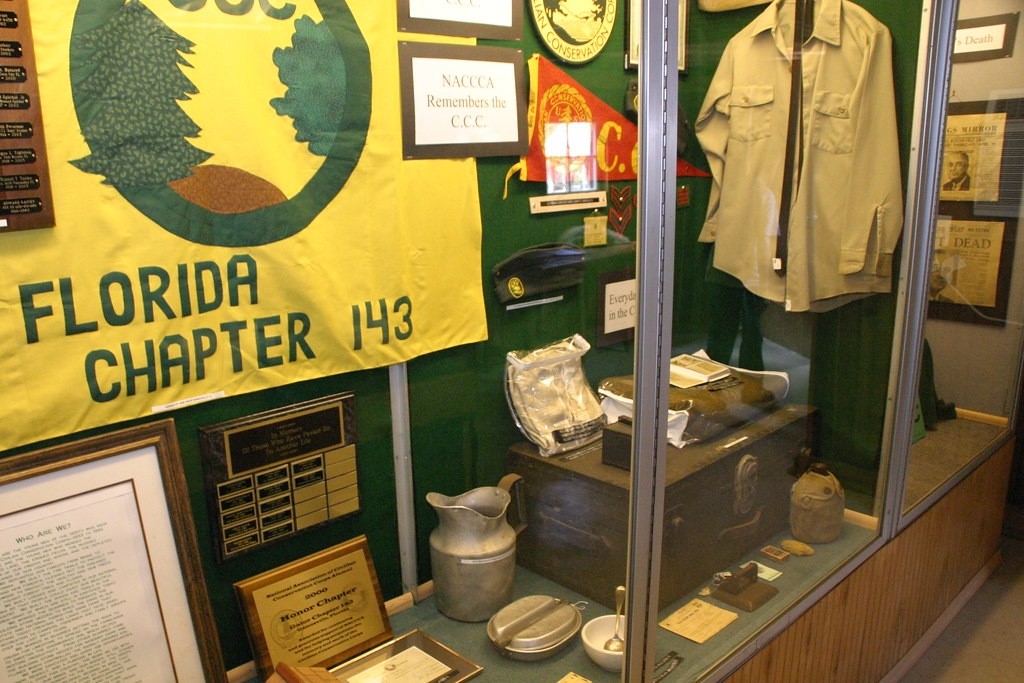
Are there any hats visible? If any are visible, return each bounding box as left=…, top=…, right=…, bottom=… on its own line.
left=491, top=239, right=585, bottom=303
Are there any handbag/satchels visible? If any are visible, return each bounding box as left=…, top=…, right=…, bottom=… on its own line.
left=503, top=331, right=610, bottom=458
left=598, top=343, right=790, bottom=450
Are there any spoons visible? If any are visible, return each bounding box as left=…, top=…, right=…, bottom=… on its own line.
left=605, top=584, right=625, bottom=650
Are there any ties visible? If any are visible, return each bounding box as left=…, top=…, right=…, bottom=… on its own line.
left=774, top=1, right=814, bottom=275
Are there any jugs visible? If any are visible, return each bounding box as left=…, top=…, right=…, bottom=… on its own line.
left=425, top=473, right=529, bottom=623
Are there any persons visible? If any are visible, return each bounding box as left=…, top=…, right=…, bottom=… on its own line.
left=929, top=248, right=956, bottom=305
left=941, top=150, right=970, bottom=191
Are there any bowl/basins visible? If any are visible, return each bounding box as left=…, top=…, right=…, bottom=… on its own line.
left=581, top=614, right=623, bottom=673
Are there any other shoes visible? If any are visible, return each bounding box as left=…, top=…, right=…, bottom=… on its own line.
left=742, top=386, right=778, bottom=406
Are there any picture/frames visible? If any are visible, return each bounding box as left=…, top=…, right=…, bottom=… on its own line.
left=927, top=216, right=1019, bottom=325
left=936, top=98, right=1024, bottom=220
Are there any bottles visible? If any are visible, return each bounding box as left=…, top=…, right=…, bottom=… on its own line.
left=789, top=462, right=845, bottom=542
